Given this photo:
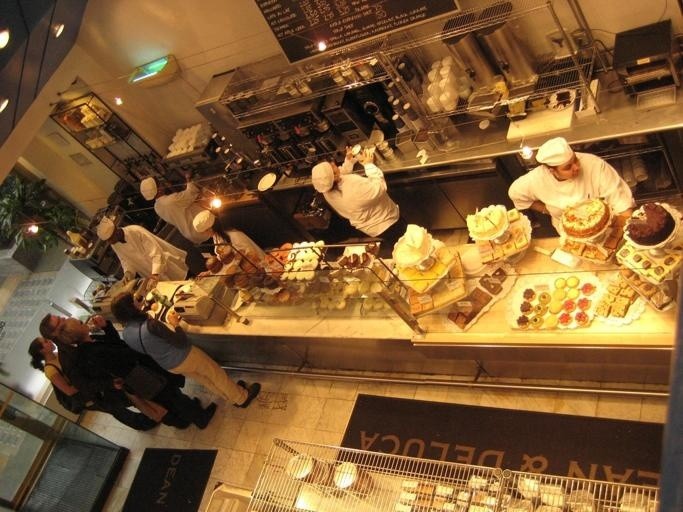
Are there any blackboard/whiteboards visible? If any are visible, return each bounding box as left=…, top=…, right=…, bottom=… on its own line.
left=254, top=0, right=462, bottom=67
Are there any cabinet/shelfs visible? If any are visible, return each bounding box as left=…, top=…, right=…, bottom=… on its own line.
left=50, top=92, right=170, bottom=193
left=246, top=439, right=501, bottom=512
left=499, top=469, right=660, bottom=512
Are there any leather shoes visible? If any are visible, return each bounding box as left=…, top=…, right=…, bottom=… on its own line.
left=191, top=397, right=216, bottom=429
left=232, top=380, right=261, bottom=408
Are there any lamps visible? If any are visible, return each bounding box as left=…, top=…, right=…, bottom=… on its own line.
left=129, top=55, right=179, bottom=89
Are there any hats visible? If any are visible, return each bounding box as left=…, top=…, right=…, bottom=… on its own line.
left=96, top=218, right=114, bottom=241
left=192, top=210, right=215, bottom=233
left=311, top=162, right=333, bottom=193
left=140, top=178, right=157, bottom=200
left=536, top=137, right=574, bottom=167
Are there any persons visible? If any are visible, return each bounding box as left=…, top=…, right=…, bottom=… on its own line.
left=39, top=313, right=134, bottom=407
left=193, top=211, right=265, bottom=276
left=94, top=219, right=195, bottom=289
left=110, top=293, right=259, bottom=408
left=309, top=144, right=406, bottom=247
left=28, top=337, right=158, bottom=429
left=506, top=136, right=637, bottom=235
left=139, top=170, right=200, bottom=246
left=57, top=315, right=216, bottom=430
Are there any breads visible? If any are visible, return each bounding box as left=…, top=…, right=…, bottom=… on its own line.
left=619, top=264, right=672, bottom=309
left=432, top=245, right=455, bottom=276
left=478, top=239, right=516, bottom=264
left=279, top=240, right=326, bottom=282
left=604, top=214, right=626, bottom=249
left=234, top=249, right=279, bottom=290
left=596, top=275, right=640, bottom=318
left=395, top=263, right=438, bottom=293
left=561, top=239, right=613, bottom=261
left=507, top=207, right=529, bottom=251
left=617, top=243, right=682, bottom=282
left=447, top=267, right=507, bottom=329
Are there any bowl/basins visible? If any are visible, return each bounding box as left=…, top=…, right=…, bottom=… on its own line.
left=425, top=56, right=470, bottom=114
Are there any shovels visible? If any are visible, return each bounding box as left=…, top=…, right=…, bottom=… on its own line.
left=534, top=246, right=582, bottom=270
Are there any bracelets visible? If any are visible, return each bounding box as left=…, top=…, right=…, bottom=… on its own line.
left=541, top=203, right=545, bottom=215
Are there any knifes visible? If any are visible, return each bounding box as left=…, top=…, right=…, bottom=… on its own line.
left=531, top=247, right=581, bottom=268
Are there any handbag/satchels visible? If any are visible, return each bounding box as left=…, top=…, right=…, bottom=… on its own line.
left=51, top=383, right=85, bottom=415
left=124, top=363, right=167, bottom=401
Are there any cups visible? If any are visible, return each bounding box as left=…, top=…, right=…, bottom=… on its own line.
left=305, top=148, right=318, bottom=164
left=387, top=61, right=425, bottom=132
left=63, top=242, right=94, bottom=259
left=211, top=131, right=246, bottom=169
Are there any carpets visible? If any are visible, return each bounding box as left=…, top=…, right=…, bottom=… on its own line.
left=120, top=448, right=218, bottom=512
left=337, top=393, right=666, bottom=512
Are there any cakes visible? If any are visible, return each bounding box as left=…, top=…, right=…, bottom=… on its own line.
left=466, top=208, right=505, bottom=237
left=205, top=257, right=222, bottom=274
left=404, top=223, right=431, bottom=257
left=335, top=241, right=377, bottom=266
left=518, top=276, right=596, bottom=328
left=561, top=198, right=610, bottom=238
left=216, top=245, right=234, bottom=265
left=629, top=202, right=675, bottom=245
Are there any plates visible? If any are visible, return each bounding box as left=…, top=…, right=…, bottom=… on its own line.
left=257, top=174, right=277, bottom=191
left=140, top=285, right=192, bottom=324
left=548, top=89, right=576, bottom=111
